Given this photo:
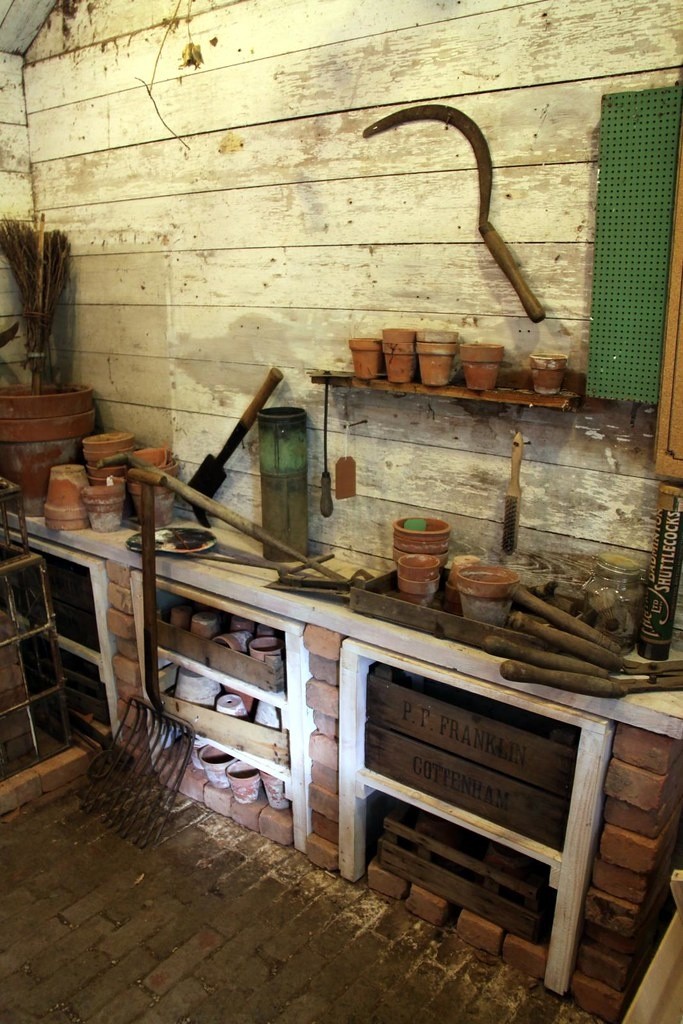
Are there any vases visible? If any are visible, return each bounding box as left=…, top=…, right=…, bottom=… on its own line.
left=391, top=514, right=522, bottom=629
left=152, top=603, right=294, bottom=808
left=347, top=325, right=570, bottom=398
left=0, top=379, right=181, bottom=532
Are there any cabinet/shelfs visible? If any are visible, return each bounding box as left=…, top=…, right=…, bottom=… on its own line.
left=127, top=569, right=312, bottom=857
left=0, top=526, right=124, bottom=748
left=336, top=636, right=615, bottom=999
